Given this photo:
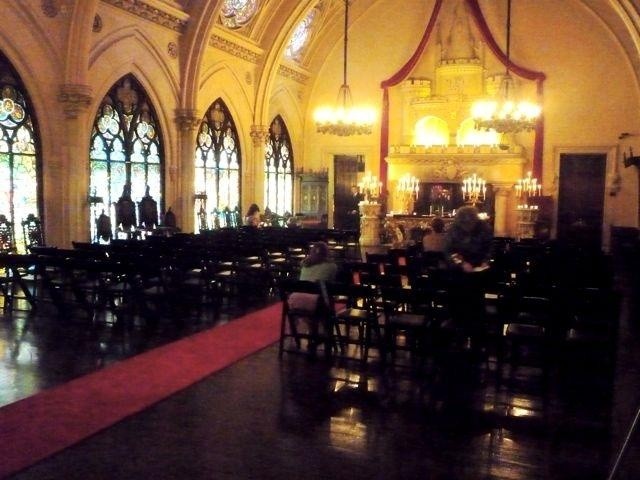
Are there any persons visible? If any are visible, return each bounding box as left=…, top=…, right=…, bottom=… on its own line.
left=345, top=185, right=364, bottom=243
left=423, top=217, right=450, bottom=270
left=444, top=206, right=494, bottom=351
left=244, top=203, right=261, bottom=227
left=300, top=240, right=338, bottom=347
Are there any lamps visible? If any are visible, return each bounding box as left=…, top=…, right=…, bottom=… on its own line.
left=473, top=0, right=542, bottom=135
left=313, top=0, right=373, bottom=137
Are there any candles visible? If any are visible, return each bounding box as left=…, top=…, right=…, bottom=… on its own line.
left=514, top=173, right=543, bottom=199
left=396, top=175, right=420, bottom=201
left=358, top=172, right=383, bottom=197
left=461, top=173, right=486, bottom=203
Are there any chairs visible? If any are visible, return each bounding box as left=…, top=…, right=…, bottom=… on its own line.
left=0, top=226, right=611, bottom=437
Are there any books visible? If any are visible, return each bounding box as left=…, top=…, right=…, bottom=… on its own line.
left=472, top=265, right=489, bottom=272
